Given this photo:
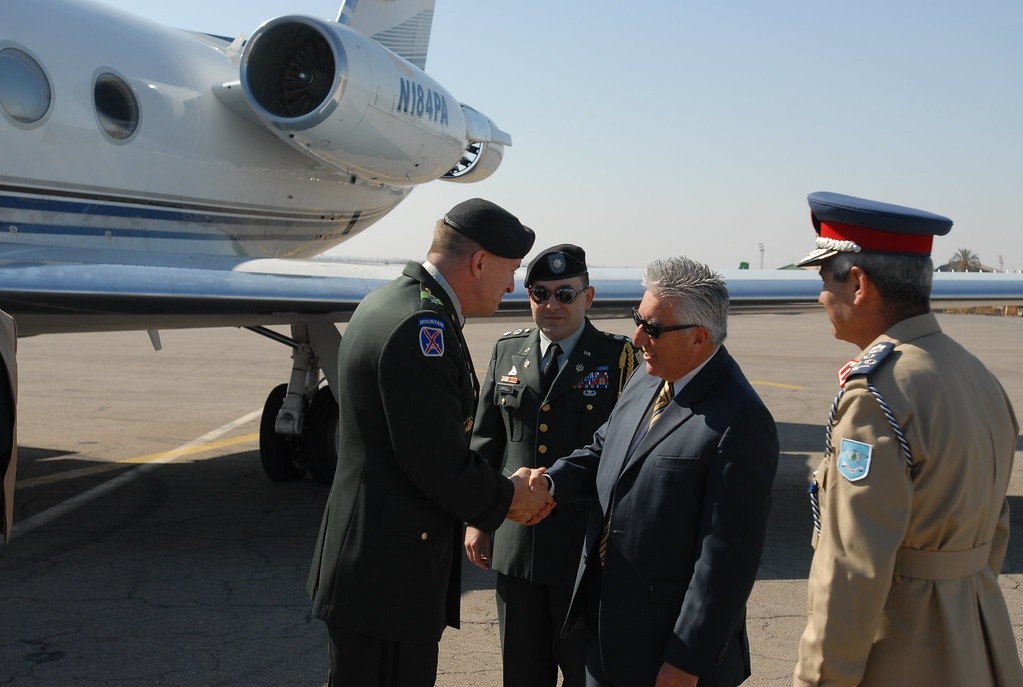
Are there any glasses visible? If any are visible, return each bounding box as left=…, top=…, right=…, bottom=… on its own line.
left=528, top=285, right=588, bottom=304
left=632, top=304, right=701, bottom=338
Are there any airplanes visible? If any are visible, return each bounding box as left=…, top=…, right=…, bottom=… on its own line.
left=0, top=0, right=1023, bottom=558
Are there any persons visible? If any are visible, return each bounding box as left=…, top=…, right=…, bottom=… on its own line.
left=777, top=190, right=1023, bottom=687
left=305, top=197, right=780, bottom=687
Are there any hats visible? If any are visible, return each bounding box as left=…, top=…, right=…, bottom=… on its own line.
left=444, top=198, right=536, bottom=259
left=524, top=244, right=588, bottom=289
left=796, top=191, right=954, bottom=267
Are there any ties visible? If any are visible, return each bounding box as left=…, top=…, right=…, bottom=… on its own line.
left=600, top=380, right=675, bottom=571
left=542, top=343, right=562, bottom=390
